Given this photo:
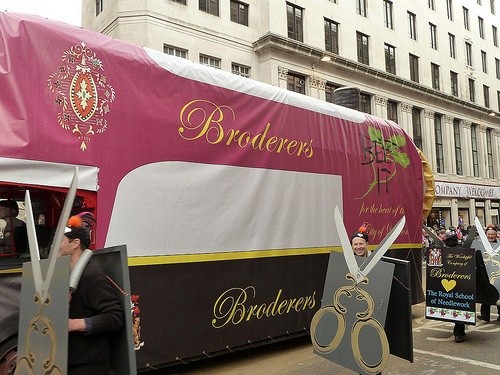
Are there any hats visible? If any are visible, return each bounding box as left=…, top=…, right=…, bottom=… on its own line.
left=351, top=226, right=368, bottom=242
left=62, top=212, right=96, bottom=247
left=485, top=224, right=497, bottom=234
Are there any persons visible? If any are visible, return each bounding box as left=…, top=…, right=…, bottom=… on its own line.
left=58, top=211, right=125, bottom=375
left=0, top=200, right=31, bottom=258
left=422, top=225, right=500, bottom=343
left=351, top=227, right=372, bottom=258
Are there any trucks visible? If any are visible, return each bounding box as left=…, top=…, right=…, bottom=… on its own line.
left=0, top=10, right=415, bottom=375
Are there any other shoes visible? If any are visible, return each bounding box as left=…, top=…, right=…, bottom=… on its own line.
left=477, top=315, right=491, bottom=322
left=455, top=336, right=466, bottom=342
left=497, top=315, right=500, bottom=321
